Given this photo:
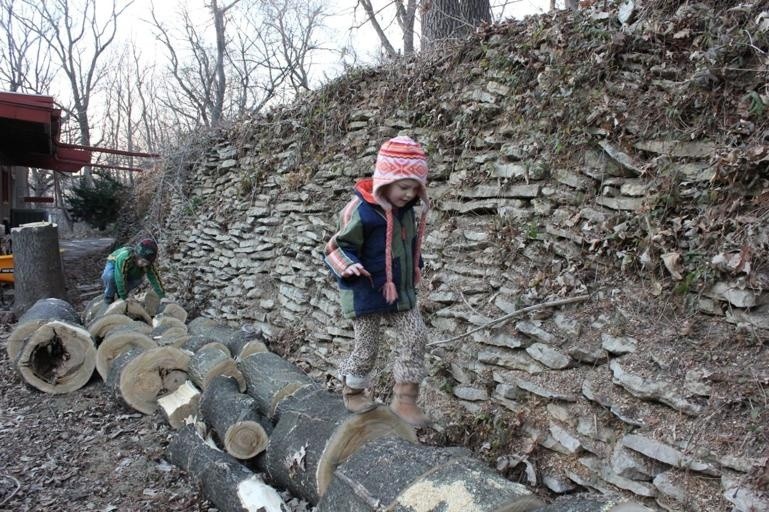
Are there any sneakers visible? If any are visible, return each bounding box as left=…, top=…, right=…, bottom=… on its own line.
left=104, top=295, right=115, bottom=304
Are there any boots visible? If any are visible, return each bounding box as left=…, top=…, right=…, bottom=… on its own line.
left=389, top=380, right=433, bottom=425
left=343, top=382, right=377, bottom=416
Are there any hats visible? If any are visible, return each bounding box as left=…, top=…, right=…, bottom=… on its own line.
left=373, top=134, right=428, bottom=212
left=134, top=238, right=158, bottom=263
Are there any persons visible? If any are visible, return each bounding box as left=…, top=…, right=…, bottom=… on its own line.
left=323, top=135, right=431, bottom=426
left=102, top=238, right=171, bottom=305
